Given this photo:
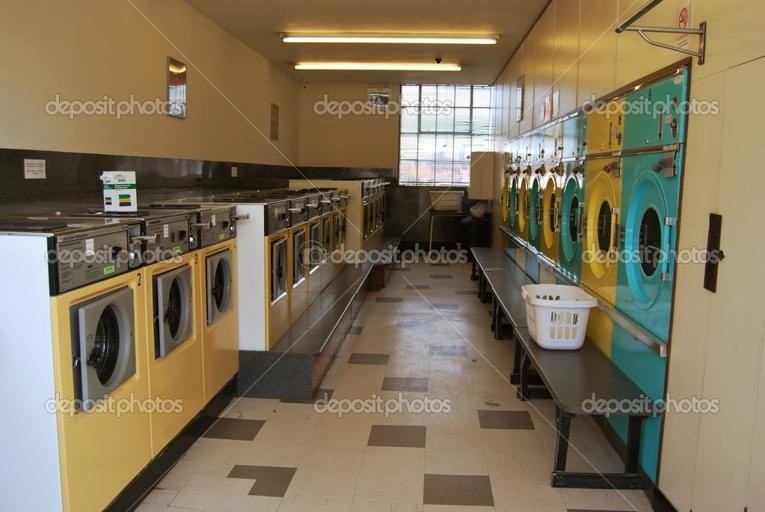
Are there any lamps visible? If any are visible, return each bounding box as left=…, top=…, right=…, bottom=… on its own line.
left=287, top=63, right=465, bottom=73
left=278, top=29, right=505, bottom=46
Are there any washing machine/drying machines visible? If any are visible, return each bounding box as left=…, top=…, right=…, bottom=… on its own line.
left=501, top=64, right=694, bottom=488
left=0, top=178, right=391, bottom=512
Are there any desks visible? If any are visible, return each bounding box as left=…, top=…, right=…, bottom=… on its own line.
left=426, top=210, right=492, bottom=260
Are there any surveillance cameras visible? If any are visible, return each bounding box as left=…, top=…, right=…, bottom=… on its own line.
left=435, top=57, right=442, bottom=63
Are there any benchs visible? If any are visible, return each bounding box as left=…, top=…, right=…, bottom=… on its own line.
left=492, top=286, right=574, bottom=385
left=481, top=260, right=537, bottom=333
left=470, top=246, right=515, bottom=298
left=512, top=325, right=660, bottom=490
